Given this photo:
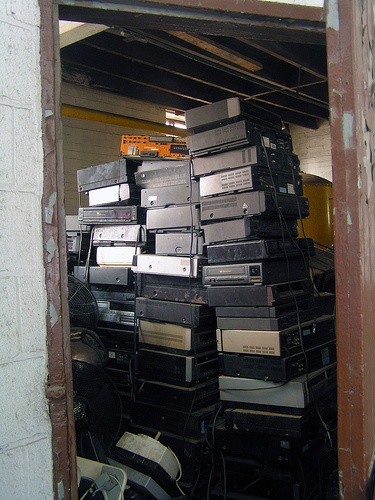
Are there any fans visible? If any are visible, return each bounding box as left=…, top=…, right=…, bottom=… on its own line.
left=69, top=327, right=108, bottom=371
left=71, top=360, right=124, bottom=466
left=67, top=274, right=99, bottom=332
